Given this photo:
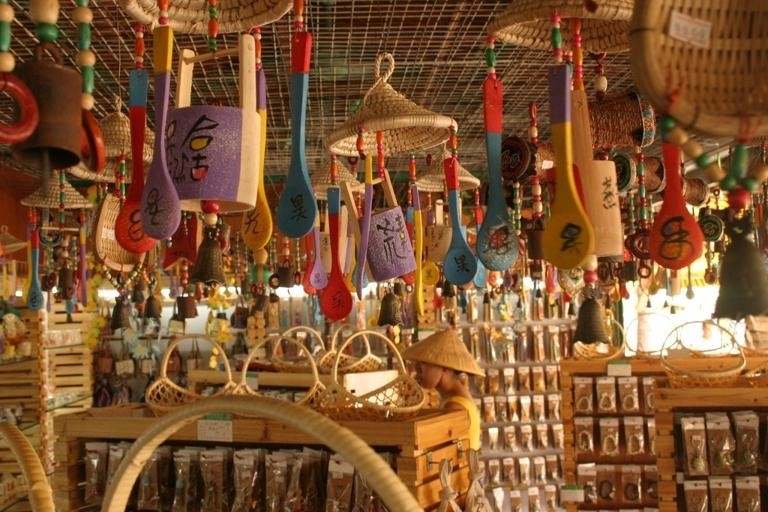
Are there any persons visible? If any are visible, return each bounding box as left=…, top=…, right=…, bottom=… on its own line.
left=403, top=325, right=490, bottom=453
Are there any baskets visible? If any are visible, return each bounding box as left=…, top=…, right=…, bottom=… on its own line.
left=145, top=333, right=237, bottom=418
left=320, top=324, right=384, bottom=374
left=572, top=313, right=768, bottom=388
left=312, top=330, right=426, bottom=418
left=232, top=336, right=326, bottom=404
left=272, top=325, right=326, bottom=373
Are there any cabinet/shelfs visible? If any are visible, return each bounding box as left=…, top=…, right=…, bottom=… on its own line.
left=558, top=353, right=768, bottom=512
left=53, top=405, right=472, bottom=512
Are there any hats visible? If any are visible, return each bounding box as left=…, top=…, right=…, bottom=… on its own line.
left=66, top=112, right=155, bottom=184
left=325, top=83, right=458, bottom=156
left=40, top=216, right=80, bottom=232
left=487, top=0, right=768, bottom=139
left=119, top=0, right=294, bottom=35
left=311, top=160, right=366, bottom=201
left=0, top=233, right=27, bottom=256
left=19, top=169, right=94, bottom=210
left=400, top=327, right=487, bottom=378
left=409, top=151, right=480, bottom=192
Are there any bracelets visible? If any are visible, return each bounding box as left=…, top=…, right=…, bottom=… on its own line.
left=577, top=392, right=659, bottom=503
